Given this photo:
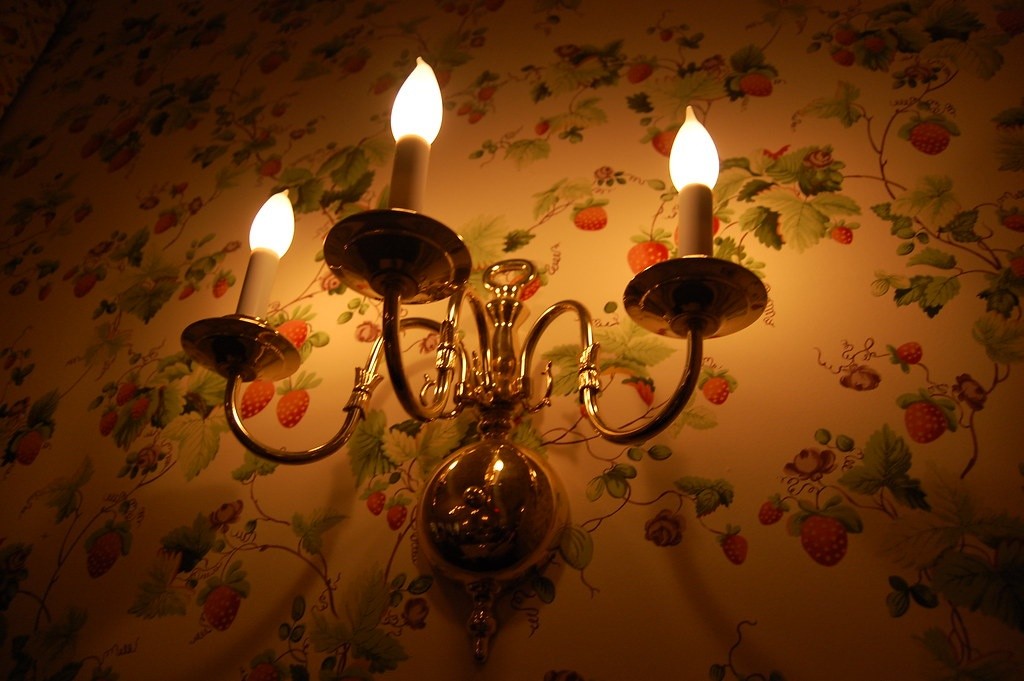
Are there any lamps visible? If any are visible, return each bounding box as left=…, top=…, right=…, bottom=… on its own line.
left=181, top=57, right=768, bottom=664
